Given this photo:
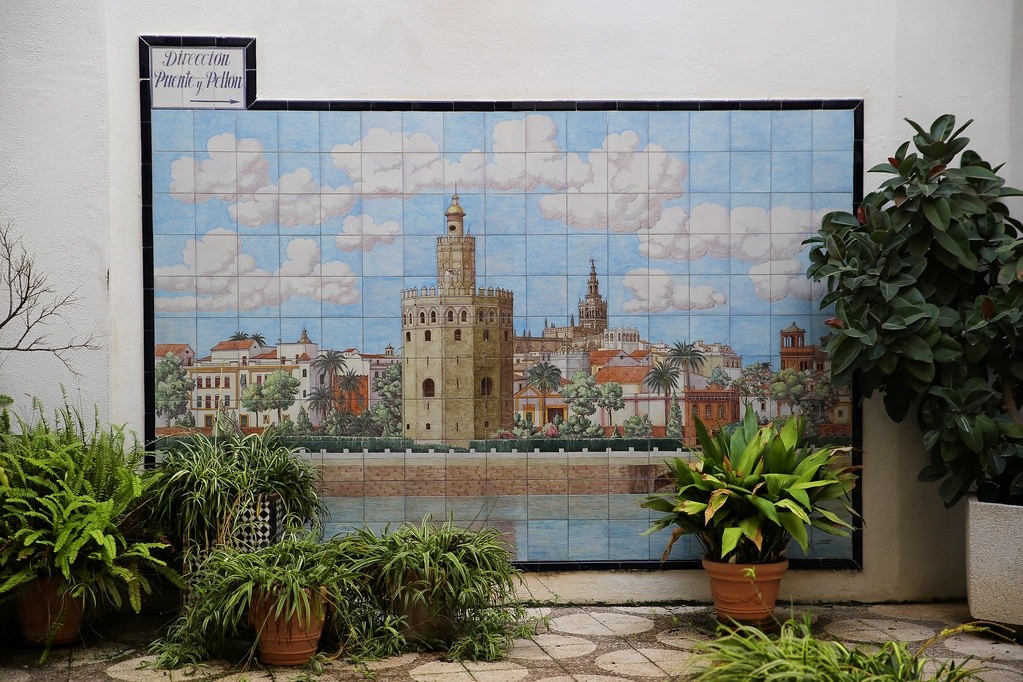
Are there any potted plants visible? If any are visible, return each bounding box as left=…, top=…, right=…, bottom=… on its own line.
left=0, top=360, right=573, bottom=682
left=799, top=111, right=1023, bottom=630
left=639, top=400, right=871, bottom=636
